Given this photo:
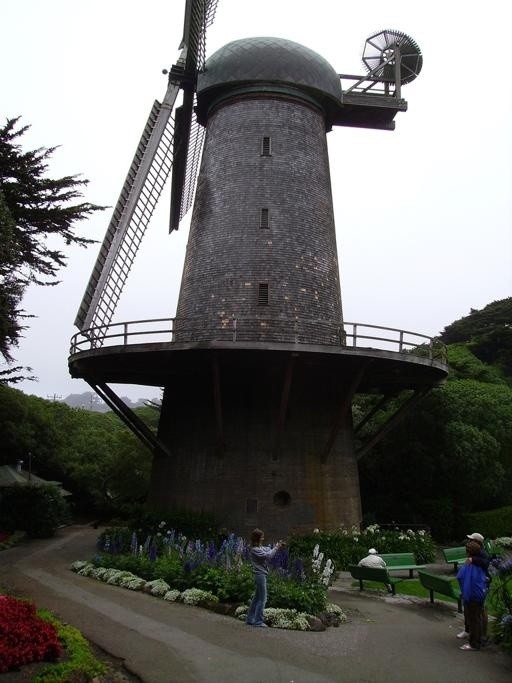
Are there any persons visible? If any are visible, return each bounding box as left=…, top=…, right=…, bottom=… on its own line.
left=356, top=548, right=393, bottom=592
left=456, top=628, right=471, bottom=638
left=207, top=527, right=230, bottom=551
left=464, top=531, right=490, bottom=645
left=455, top=540, right=487, bottom=651
left=242, top=527, right=285, bottom=628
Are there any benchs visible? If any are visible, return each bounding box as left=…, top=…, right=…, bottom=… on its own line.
left=347, top=535, right=511, bottom=616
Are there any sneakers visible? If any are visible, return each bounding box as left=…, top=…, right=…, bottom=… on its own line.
left=460, top=644, right=478, bottom=650
left=457, top=631, right=470, bottom=639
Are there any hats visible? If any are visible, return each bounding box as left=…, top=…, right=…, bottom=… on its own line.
left=467, top=533, right=484, bottom=542
left=369, top=549, right=378, bottom=554
